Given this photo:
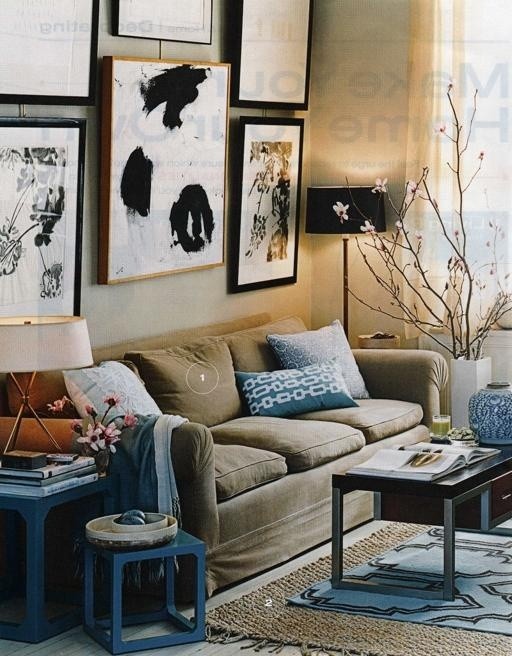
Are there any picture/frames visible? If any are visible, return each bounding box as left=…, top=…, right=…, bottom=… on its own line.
left=98, top=56, right=231, bottom=285
left=0, top=117, right=86, bottom=316
left=111, top=0, right=213, bottom=45
left=0, top=0, right=99, bottom=106
left=228, top=117, right=304, bottom=293
left=230, top=0, right=314, bottom=111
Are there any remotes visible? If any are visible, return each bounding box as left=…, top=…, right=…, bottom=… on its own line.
left=46, top=452, right=79, bottom=465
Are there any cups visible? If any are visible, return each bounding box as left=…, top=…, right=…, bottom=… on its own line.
left=432, top=414, right=450, bottom=434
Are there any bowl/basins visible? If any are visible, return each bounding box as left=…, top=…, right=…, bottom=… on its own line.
left=84, top=513, right=177, bottom=552
left=111, top=514, right=167, bottom=533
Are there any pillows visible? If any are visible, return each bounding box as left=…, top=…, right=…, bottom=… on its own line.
left=63, top=359, right=164, bottom=426
left=137, top=340, right=240, bottom=426
left=265, top=318, right=370, bottom=398
left=232, top=361, right=360, bottom=418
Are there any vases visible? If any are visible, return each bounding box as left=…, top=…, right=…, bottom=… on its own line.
left=451, top=357, right=492, bottom=428
left=80, top=451, right=111, bottom=477
left=469, top=382, right=512, bottom=444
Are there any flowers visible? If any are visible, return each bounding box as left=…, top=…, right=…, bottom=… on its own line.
left=332, top=78, right=512, bottom=360
left=47, top=394, right=138, bottom=453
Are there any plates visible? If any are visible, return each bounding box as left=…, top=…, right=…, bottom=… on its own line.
left=447, top=435, right=479, bottom=445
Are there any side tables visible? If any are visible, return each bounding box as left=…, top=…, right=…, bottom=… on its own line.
left=0, top=472, right=120, bottom=644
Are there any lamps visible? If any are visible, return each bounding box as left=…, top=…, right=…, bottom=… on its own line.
left=0, top=316, right=94, bottom=453
left=305, top=186, right=386, bottom=339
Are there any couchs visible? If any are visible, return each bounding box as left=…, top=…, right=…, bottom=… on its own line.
left=1, top=312, right=451, bottom=600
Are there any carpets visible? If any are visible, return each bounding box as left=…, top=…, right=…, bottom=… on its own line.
left=190, top=519, right=512, bottom=656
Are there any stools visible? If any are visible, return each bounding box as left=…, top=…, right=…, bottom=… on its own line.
left=83, top=528, right=206, bottom=655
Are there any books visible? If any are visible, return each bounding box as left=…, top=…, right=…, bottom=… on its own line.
left=346, top=441, right=502, bottom=483
left=1, top=457, right=99, bottom=498
